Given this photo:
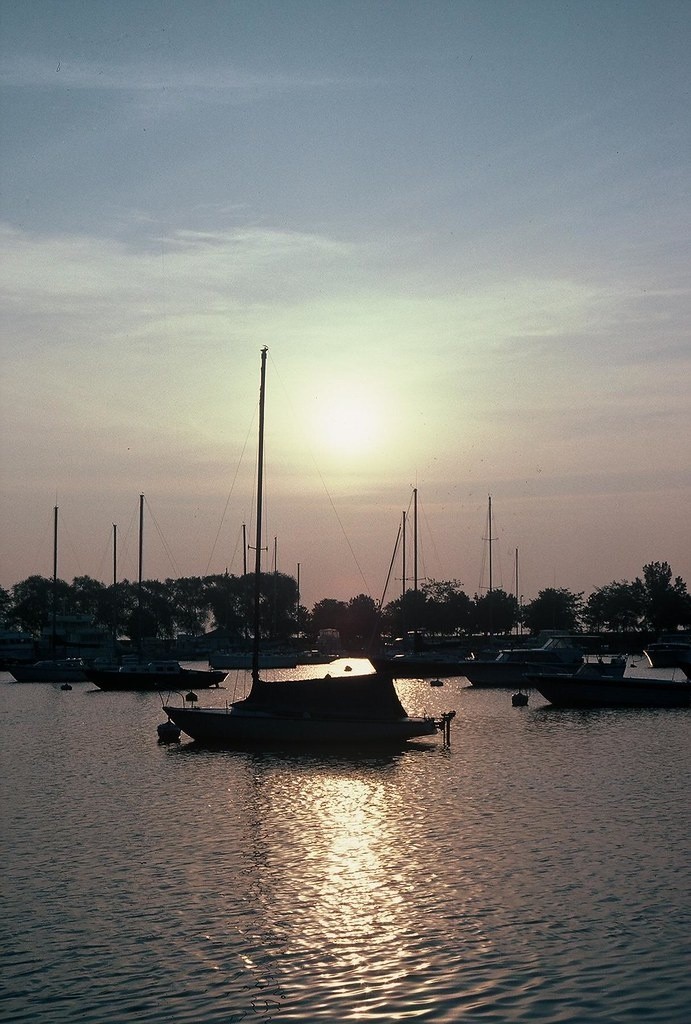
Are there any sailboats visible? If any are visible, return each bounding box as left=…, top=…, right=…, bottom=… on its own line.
left=2, top=475, right=690, bottom=711
left=160, top=345, right=456, bottom=763
